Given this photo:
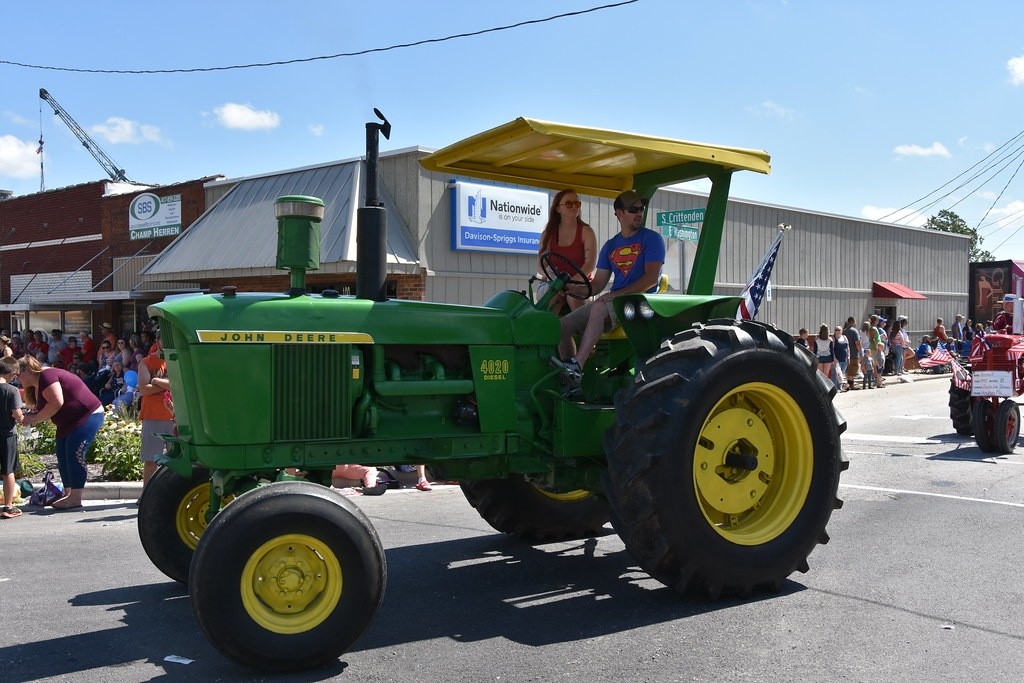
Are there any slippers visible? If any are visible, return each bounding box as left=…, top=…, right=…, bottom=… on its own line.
left=416, top=482, right=434, bottom=491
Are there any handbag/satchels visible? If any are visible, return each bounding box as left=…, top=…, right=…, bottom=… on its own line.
left=30, top=471, right=65, bottom=506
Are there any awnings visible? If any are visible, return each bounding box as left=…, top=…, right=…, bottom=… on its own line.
left=872, top=281, right=927, bottom=300
left=136, top=161, right=420, bottom=281
left=29, top=288, right=210, bottom=300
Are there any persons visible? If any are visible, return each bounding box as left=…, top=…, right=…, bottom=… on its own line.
left=537, top=189, right=597, bottom=362
left=917, top=335, right=944, bottom=374
left=932, top=318, right=948, bottom=342
left=832, top=325, right=851, bottom=392
left=136, top=329, right=178, bottom=503
left=814, top=324, right=834, bottom=377
left=551, top=189, right=663, bottom=377
left=973, top=320, right=993, bottom=338
left=0, top=317, right=160, bottom=418
left=989, top=293, right=1024, bottom=335
left=943, top=314, right=975, bottom=362
left=796, top=328, right=810, bottom=349
left=0, top=355, right=25, bottom=517
left=12, top=355, right=105, bottom=507
left=842, top=314, right=915, bottom=389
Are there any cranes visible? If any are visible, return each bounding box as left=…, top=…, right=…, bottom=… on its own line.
left=38, top=87, right=161, bottom=188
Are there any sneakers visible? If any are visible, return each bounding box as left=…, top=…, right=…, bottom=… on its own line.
left=1, top=506, right=23, bottom=519
left=549, top=356, right=584, bottom=386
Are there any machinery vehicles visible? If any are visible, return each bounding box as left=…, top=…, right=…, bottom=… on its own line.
left=136, top=107, right=852, bottom=679
left=948, top=274, right=1024, bottom=455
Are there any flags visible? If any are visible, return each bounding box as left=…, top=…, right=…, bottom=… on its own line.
left=971, top=334, right=990, bottom=356
left=735, top=231, right=784, bottom=321
left=918, top=340, right=972, bottom=391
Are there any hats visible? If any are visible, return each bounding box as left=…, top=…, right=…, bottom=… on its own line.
left=956, top=315, right=965, bottom=320
left=52, top=329, right=62, bottom=333
left=99, top=323, right=116, bottom=330
left=898, top=316, right=908, bottom=322
left=879, top=318, right=887, bottom=323
left=1004, top=294, right=1016, bottom=302
left=613, top=190, right=651, bottom=211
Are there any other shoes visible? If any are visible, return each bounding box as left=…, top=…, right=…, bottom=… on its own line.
left=52, top=498, right=83, bottom=509
left=363, top=484, right=387, bottom=495
left=837, top=384, right=884, bottom=393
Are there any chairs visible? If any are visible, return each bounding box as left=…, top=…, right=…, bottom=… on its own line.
left=598, top=274, right=668, bottom=340
left=100, top=387, right=140, bottom=418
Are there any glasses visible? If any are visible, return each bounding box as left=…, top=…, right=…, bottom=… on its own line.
left=617, top=206, right=647, bottom=214
left=559, top=201, right=582, bottom=209
left=117, top=342, right=124, bottom=345
left=102, top=346, right=110, bottom=349
